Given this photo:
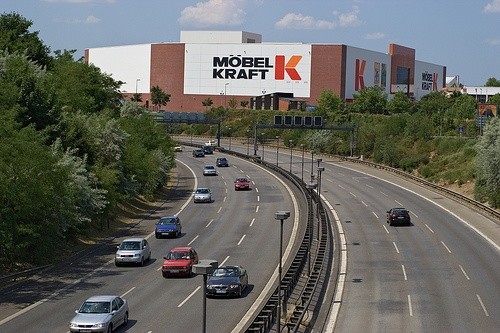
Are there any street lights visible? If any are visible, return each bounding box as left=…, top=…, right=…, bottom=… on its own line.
left=228, top=127, right=280, bottom=167
left=136, top=79, right=140, bottom=98
left=220, top=83, right=229, bottom=106
left=192, top=259, right=218, bottom=333
left=300, top=144, right=316, bottom=183
left=315, top=158, right=324, bottom=240
left=306, top=183, right=318, bottom=274
left=289, top=140, right=293, bottom=172
left=274, top=210, right=290, bottom=333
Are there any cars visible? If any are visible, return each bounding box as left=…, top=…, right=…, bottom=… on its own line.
left=193, top=188, right=211, bottom=203
left=115, top=238, right=151, bottom=266
left=69, top=295, right=129, bottom=333
left=234, top=177, right=250, bottom=191
left=386, top=207, right=411, bottom=226
left=161, top=247, right=198, bottom=278
left=155, top=215, right=181, bottom=238
left=203, top=158, right=229, bottom=176
left=174, top=144, right=181, bottom=152
left=207, top=265, right=247, bottom=298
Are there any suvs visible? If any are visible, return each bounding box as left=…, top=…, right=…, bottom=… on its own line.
left=192, top=146, right=213, bottom=157
